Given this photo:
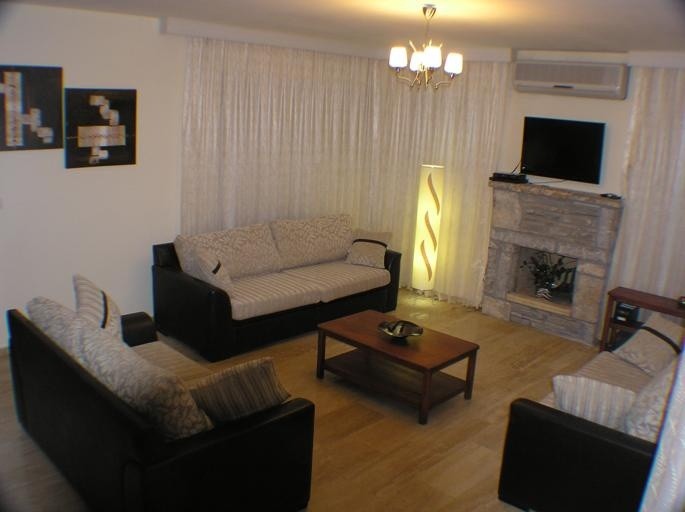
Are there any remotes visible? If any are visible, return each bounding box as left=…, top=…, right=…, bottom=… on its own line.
left=600, top=193, right=621, bottom=200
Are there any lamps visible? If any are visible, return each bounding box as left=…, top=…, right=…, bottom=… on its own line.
left=387, top=3, right=464, bottom=90
left=411, top=162, right=446, bottom=290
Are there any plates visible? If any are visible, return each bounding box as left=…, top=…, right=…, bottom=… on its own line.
left=377, top=320, right=424, bottom=344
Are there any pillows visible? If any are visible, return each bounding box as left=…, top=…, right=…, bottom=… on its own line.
left=27, top=296, right=116, bottom=362
left=552, top=311, right=685, bottom=444
left=187, top=352, right=290, bottom=426
left=76, top=326, right=206, bottom=442
left=173, top=210, right=392, bottom=294
left=70, top=272, right=126, bottom=347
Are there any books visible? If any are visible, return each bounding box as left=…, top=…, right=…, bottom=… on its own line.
left=344, top=357, right=377, bottom=377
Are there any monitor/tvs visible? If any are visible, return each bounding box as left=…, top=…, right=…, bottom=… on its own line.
left=520, top=115, right=606, bottom=185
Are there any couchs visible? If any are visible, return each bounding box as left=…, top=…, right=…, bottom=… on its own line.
left=5, top=305, right=315, bottom=510
left=498, top=319, right=685, bottom=512
left=152, top=214, right=404, bottom=362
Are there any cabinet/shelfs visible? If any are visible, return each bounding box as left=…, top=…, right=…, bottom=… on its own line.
left=600, top=286, right=685, bottom=352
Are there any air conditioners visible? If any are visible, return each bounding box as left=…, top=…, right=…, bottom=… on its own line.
left=511, top=61, right=630, bottom=100
